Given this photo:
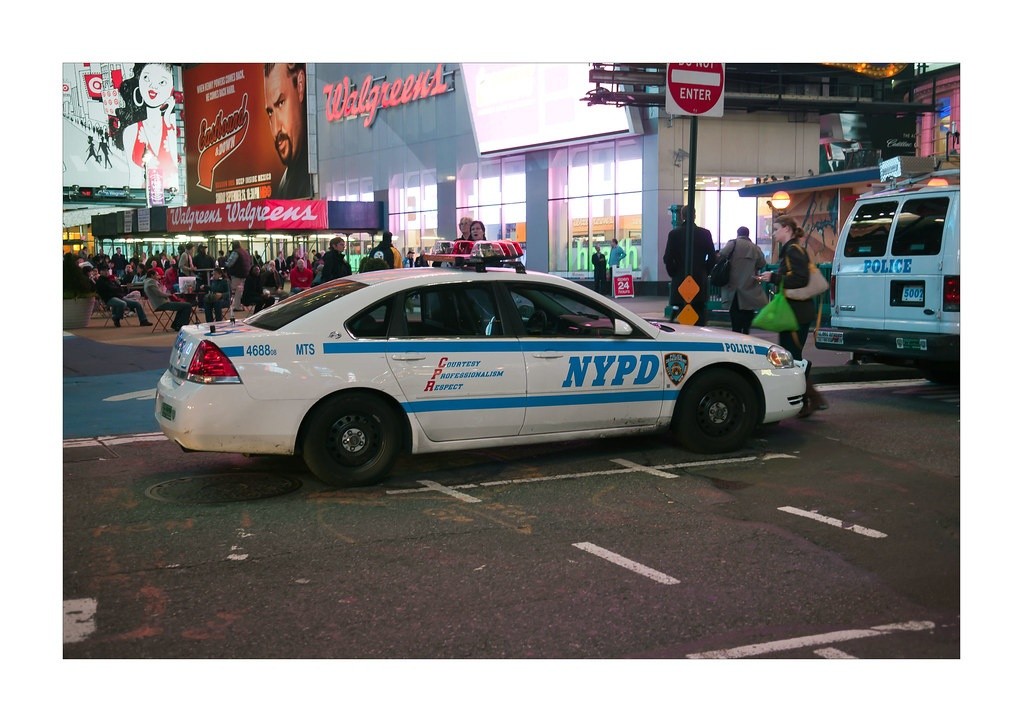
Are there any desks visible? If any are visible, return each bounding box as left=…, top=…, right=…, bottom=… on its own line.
left=173, top=291, right=209, bottom=324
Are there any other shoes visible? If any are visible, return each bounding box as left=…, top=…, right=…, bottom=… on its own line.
left=126, top=311, right=135, bottom=317
left=112, top=317, right=121, bottom=327
left=171, top=324, right=181, bottom=331
left=798, top=370, right=826, bottom=418
left=140, top=319, right=153, bottom=326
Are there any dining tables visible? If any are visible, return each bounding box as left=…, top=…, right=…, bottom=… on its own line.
left=95, top=287, right=280, bottom=334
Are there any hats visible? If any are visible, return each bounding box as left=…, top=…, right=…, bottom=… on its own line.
left=98, top=265, right=110, bottom=274
left=231, top=240, right=241, bottom=245
left=407, top=251, right=415, bottom=254
left=186, top=243, right=195, bottom=250
left=197, top=245, right=207, bottom=252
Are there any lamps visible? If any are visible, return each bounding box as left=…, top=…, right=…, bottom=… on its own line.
left=72, top=185, right=129, bottom=195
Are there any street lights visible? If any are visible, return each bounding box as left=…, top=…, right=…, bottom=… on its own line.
left=771, top=190, right=791, bottom=258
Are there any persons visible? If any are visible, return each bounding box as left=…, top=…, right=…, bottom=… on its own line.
left=592, top=239, right=607, bottom=295
left=760, top=215, right=827, bottom=417
left=663, top=205, right=717, bottom=327
left=263, top=63, right=311, bottom=200
left=608, top=238, right=627, bottom=284
left=709, top=226, right=766, bottom=335
left=710, top=250, right=720, bottom=277
left=63, top=217, right=487, bottom=332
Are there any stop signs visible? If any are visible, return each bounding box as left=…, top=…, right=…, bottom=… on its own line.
left=665, top=62, right=727, bottom=120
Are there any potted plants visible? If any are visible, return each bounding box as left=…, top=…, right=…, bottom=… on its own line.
left=63, top=253, right=97, bottom=329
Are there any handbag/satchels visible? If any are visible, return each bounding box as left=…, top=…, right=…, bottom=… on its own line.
left=751, top=275, right=799, bottom=332
left=784, top=244, right=829, bottom=300
left=175, top=273, right=186, bottom=283
left=711, top=262, right=729, bottom=287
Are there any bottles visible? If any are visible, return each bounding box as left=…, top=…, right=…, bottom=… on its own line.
left=183, top=282, right=192, bottom=293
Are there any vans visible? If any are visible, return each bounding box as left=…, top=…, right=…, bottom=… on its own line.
left=812, top=187, right=961, bottom=388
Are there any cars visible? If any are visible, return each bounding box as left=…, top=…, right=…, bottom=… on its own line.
left=153, top=238, right=811, bottom=489
